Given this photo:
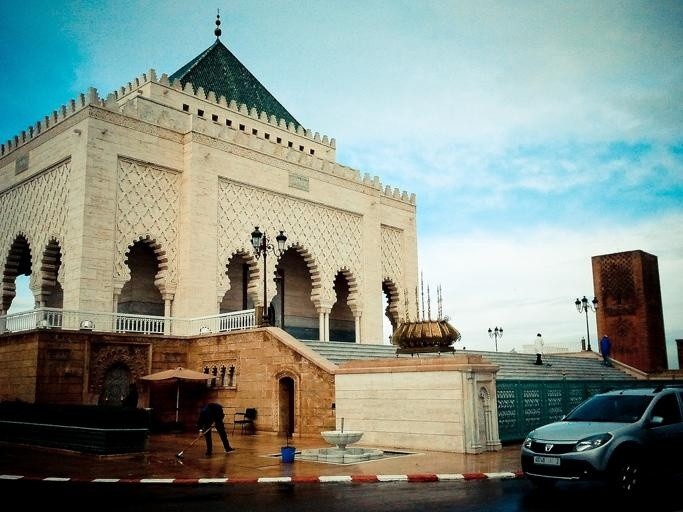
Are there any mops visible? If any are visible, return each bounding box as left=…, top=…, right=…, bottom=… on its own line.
left=175, top=426, right=214, bottom=467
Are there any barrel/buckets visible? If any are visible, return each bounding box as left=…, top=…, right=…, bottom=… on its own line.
left=281, top=446, right=296, bottom=465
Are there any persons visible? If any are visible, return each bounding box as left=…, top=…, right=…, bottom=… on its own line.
left=197, top=401, right=237, bottom=456
left=600, top=334, right=613, bottom=367
left=534, top=332, right=544, bottom=365
left=120, top=383, right=138, bottom=408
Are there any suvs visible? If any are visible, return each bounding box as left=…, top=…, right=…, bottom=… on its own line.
left=520, top=383, right=681, bottom=497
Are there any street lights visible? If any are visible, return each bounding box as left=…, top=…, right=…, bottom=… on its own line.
left=487, top=326, right=502, bottom=352
left=574, top=295, right=598, bottom=352
left=249, top=225, right=288, bottom=328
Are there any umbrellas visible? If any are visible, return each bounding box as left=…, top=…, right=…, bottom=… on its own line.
left=140, top=367, right=221, bottom=423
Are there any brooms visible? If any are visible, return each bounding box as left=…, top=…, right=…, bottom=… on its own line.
left=542, top=352, right=553, bottom=367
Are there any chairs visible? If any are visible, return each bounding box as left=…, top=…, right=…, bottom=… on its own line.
left=232, top=408, right=257, bottom=433
